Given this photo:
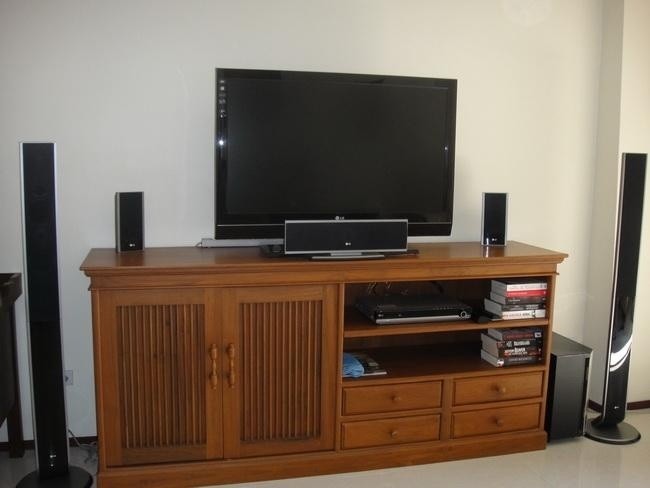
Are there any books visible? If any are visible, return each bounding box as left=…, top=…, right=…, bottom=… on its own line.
left=340, top=350, right=387, bottom=378
left=480, top=279, right=548, bottom=368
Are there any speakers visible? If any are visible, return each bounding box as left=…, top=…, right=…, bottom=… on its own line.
left=585, top=151, right=648, bottom=443
left=284, top=218, right=408, bottom=260
left=481, top=191, right=508, bottom=248
left=114, top=191, right=145, bottom=252
left=16, top=140, right=94, bottom=487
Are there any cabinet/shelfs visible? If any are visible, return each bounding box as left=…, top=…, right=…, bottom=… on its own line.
left=79, top=239, right=569, bottom=488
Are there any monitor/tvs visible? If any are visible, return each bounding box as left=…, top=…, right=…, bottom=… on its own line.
left=213, top=67, right=458, bottom=259
